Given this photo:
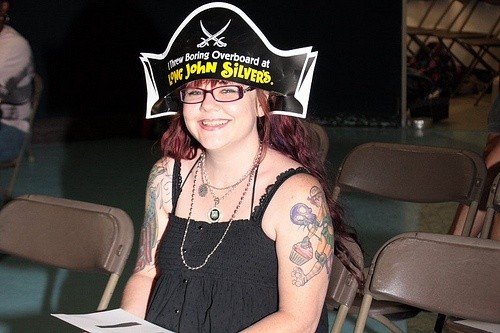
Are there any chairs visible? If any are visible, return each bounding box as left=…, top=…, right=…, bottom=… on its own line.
left=0, top=78, right=43, bottom=202
left=353, top=232, right=500, bottom=333
left=331, top=141, right=487, bottom=237
left=406, top=0, right=500, bottom=106
left=0, top=194, right=134, bottom=333
left=325, top=233, right=365, bottom=333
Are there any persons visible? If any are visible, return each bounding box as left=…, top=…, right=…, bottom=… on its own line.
left=0, top=0, right=34, bottom=163
left=453, top=94, right=500, bottom=240
left=120, top=2, right=364, bottom=333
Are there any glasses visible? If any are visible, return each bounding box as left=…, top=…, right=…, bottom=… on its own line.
left=179, top=85, right=256, bottom=104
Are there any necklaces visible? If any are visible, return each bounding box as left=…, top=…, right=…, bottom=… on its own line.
left=180, top=142, right=262, bottom=270
